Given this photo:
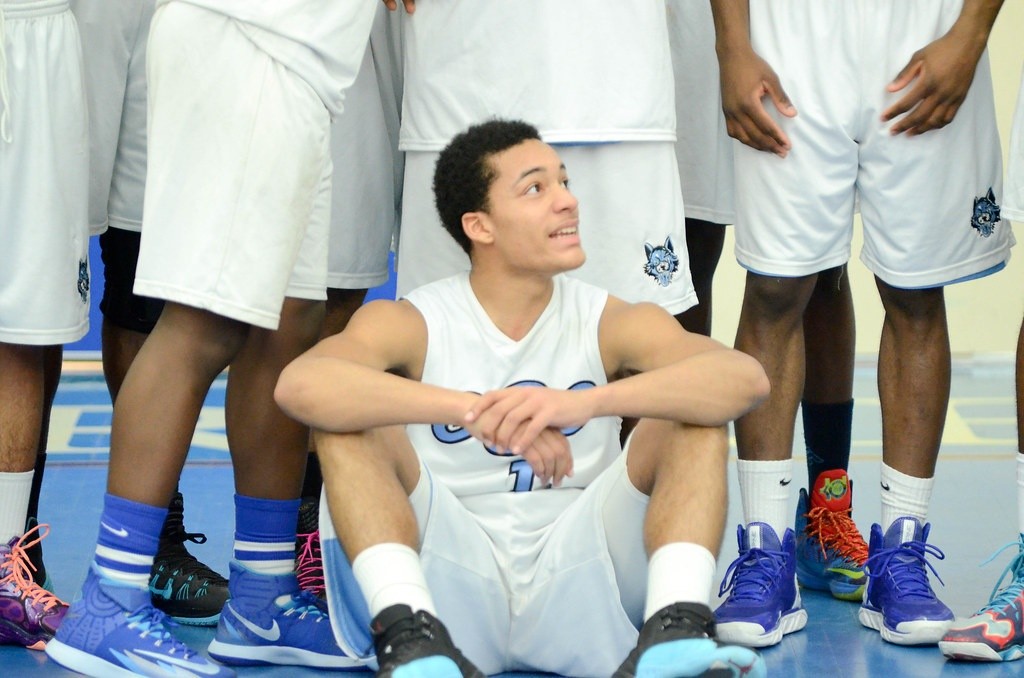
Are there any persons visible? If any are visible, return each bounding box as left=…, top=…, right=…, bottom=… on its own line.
left=274, top=117, right=771, bottom=678
left=0, top=0, right=1024, bottom=677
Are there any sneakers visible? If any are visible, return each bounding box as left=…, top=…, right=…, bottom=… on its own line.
left=45, top=565, right=237, bottom=678
left=0, top=524, right=72, bottom=651
left=208, top=560, right=370, bottom=670
left=940, top=534, right=1024, bottom=660
left=710, top=522, right=807, bottom=646
left=292, top=495, right=326, bottom=598
left=149, top=493, right=230, bottom=625
left=609, top=603, right=764, bottom=678
left=21, top=517, right=53, bottom=591
left=856, top=517, right=956, bottom=644
left=794, top=468, right=869, bottom=599
left=368, top=604, right=485, bottom=678
